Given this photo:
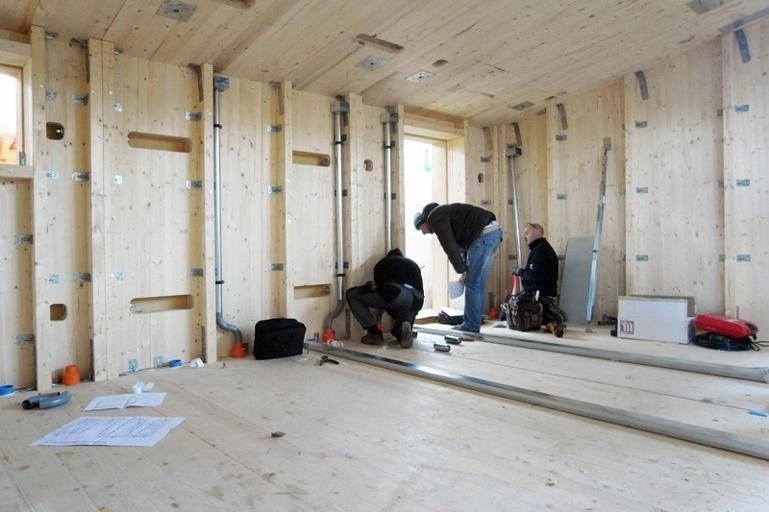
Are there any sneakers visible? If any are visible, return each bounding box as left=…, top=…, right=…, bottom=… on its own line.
left=453, top=324, right=480, bottom=332
left=549, top=302, right=568, bottom=322
left=399, top=320, right=413, bottom=348
left=361, top=329, right=384, bottom=344
left=545, top=308, right=563, bottom=324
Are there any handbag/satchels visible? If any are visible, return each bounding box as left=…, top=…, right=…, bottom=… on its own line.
left=253, top=318, right=306, bottom=359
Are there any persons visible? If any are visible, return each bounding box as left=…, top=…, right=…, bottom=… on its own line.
left=510, top=219, right=569, bottom=339
left=413, top=202, right=503, bottom=334
left=343, top=246, right=424, bottom=348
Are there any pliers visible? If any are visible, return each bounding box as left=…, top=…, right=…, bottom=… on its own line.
left=319, top=355, right=339, bottom=365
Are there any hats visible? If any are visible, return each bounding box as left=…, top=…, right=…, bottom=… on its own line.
left=413, top=212, right=424, bottom=230
